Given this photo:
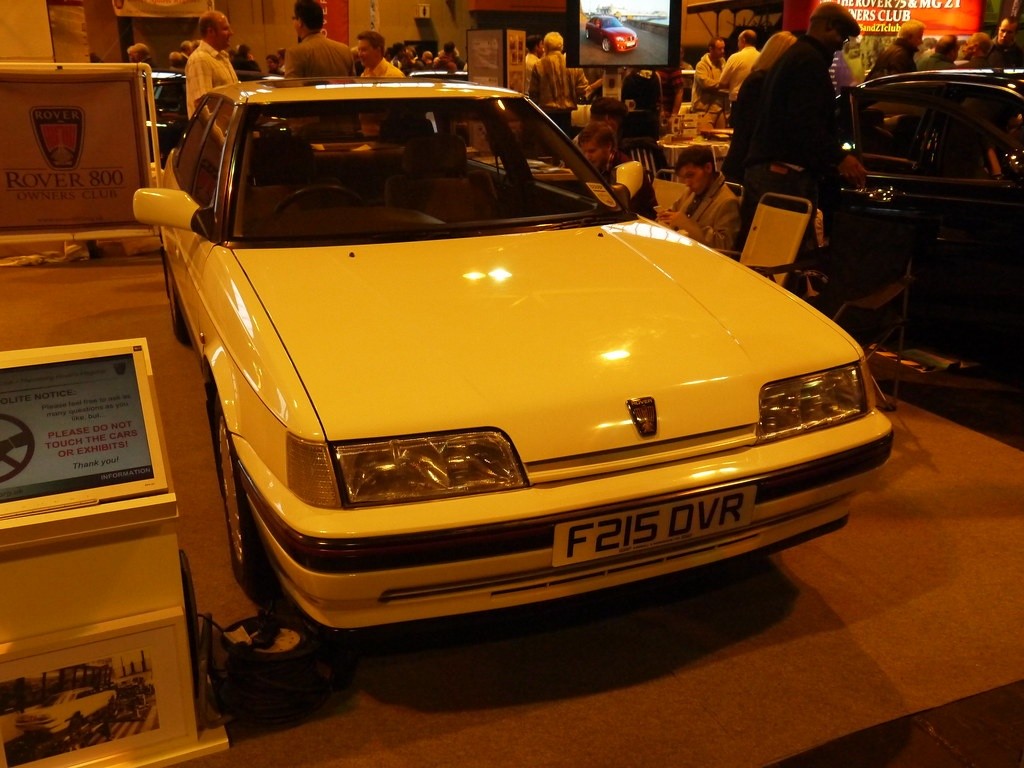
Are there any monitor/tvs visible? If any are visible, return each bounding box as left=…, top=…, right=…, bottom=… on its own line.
left=0, top=345, right=169, bottom=519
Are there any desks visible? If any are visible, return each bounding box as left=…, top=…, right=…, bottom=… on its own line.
left=656, top=135, right=732, bottom=173
left=568, top=105, right=591, bottom=126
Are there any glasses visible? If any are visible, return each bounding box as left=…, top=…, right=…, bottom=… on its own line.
left=839, top=23, right=850, bottom=44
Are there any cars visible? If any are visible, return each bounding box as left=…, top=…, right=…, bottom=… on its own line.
left=825, top=69, right=1024, bottom=377
left=132, top=70, right=894, bottom=631
left=585, top=15, right=639, bottom=52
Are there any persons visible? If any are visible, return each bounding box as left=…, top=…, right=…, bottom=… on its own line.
left=525, top=33, right=693, bottom=138
left=284, top=0, right=356, bottom=77
left=691, top=30, right=760, bottom=128
left=228, top=45, right=261, bottom=81
left=574, top=98, right=658, bottom=221
left=722, top=3, right=867, bottom=310
left=864, top=16, right=1024, bottom=83
left=266, top=49, right=285, bottom=76
left=358, top=32, right=406, bottom=78
left=352, top=47, right=364, bottom=76
left=384, top=41, right=468, bottom=75
left=169, top=40, right=200, bottom=67
left=127, top=43, right=156, bottom=67
left=656, top=145, right=742, bottom=256
left=185, top=11, right=238, bottom=121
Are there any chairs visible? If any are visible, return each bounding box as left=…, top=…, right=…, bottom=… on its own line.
left=622, top=136, right=919, bottom=412
left=386, top=132, right=501, bottom=226
left=246, top=136, right=323, bottom=211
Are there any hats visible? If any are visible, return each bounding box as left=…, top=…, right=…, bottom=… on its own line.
left=811, top=2, right=860, bottom=37
